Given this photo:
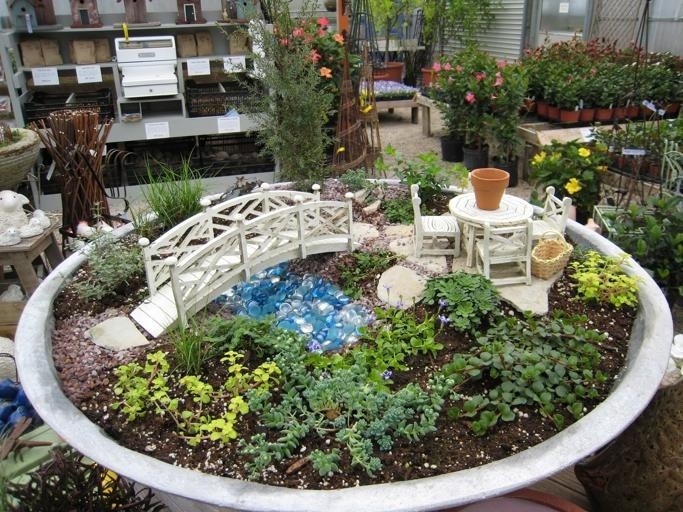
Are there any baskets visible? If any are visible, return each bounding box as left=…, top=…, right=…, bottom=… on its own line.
left=531, top=230, right=574, bottom=281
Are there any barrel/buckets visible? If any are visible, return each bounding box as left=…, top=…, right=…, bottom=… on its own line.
left=492, top=154, right=517, bottom=186
left=441, top=136, right=464, bottom=161
left=463, top=142, right=488, bottom=170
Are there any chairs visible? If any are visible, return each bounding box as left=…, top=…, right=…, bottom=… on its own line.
left=534, top=186, right=572, bottom=241
left=475, top=218, right=533, bottom=286
left=412, top=185, right=459, bottom=258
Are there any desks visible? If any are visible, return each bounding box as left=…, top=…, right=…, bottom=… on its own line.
left=361, top=100, right=420, bottom=129
left=0, top=213, right=60, bottom=342
left=448, top=194, right=533, bottom=267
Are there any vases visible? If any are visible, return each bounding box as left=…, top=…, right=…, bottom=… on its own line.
left=373, top=73, right=390, bottom=81
left=420, top=67, right=434, bottom=88
left=374, top=62, right=403, bottom=85
left=554, top=193, right=587, bottom=225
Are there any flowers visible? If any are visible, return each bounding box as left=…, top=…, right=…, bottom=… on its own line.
left=427, top=32, right=682, bottom=209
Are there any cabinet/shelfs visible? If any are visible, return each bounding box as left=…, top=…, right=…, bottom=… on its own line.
left=0, top=15, right=279, bottom=244
